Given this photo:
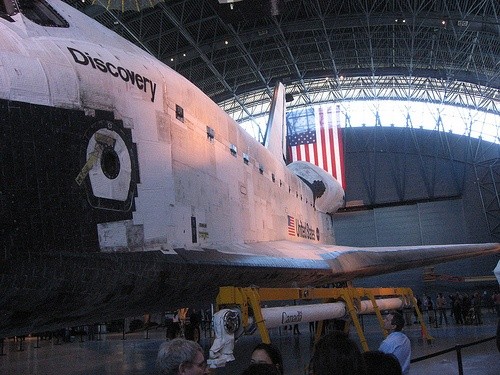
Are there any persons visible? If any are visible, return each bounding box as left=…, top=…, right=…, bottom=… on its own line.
left=156, top=292, right=484, bottom=375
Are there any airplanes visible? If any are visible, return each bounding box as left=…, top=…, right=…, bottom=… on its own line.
left=0, top=0, right=500, bottom=341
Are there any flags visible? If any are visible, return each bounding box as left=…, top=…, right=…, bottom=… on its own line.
left=286, top=105, right=346, bottom=192
